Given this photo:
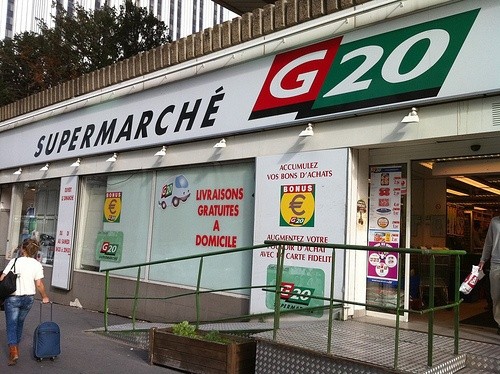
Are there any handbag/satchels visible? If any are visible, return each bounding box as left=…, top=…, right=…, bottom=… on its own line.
left=459, top=264, right=485, bottom=294
left=0, top=258, right=18, bottom=305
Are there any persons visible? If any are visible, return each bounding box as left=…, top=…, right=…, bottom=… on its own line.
left=478, top=214, right=500, bottom=335
left=0, top=238, right=50, bottom=366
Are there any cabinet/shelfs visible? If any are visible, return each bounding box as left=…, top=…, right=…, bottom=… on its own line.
left=463, top=210, right=492, bottom=254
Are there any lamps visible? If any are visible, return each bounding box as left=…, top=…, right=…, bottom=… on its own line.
left=70, top=158, right=80, bottom=167
left=214, top=138, right=226, bottom=148
left=155, top=145, right=167, bottom=156
left=402, top=107, right=419, bottom=122
left=298, top=123, right=314, bottom=137
left=40, top=163, right=49, bottom=170
left=105, top=153, right=118, bottom=162
left=13, top=168, right=22, bottom=175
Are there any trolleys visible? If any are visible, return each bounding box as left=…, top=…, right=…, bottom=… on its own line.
left=418, top=253, right=453, bottom=311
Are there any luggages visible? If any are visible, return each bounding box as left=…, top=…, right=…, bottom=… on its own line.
left=33, top=301, right=60, bottom=361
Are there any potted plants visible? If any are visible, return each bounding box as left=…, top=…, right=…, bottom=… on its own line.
left=149, top=319, right=256, bottom=374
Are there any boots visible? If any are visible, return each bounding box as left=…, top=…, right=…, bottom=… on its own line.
left=7, top=344, right=19, bottom=365
